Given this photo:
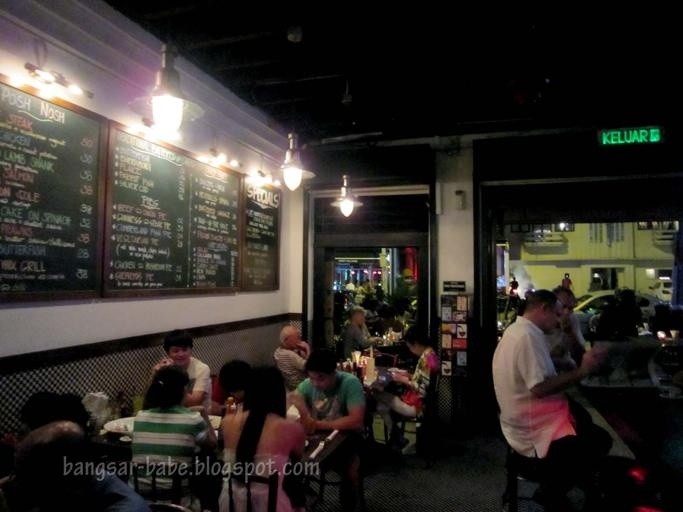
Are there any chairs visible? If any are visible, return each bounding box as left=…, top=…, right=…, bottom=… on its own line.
left=388, top=367, right=442, bottom=455
left=228, top=469, right=279, bottom=512
left=375, top=352, right=398, bottom=366
left=132, top=461, right=182, bottom=505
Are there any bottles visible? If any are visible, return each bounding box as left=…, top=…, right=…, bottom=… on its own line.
left=333, top=323, right=399, bottom=384
left=223, top=395, right=235, bottom=416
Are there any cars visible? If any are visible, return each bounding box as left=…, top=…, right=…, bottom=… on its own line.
left=570, top=289, right=666, bottom=334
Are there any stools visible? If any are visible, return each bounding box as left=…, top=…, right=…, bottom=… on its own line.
left=501, top=442, right=552, bottom=512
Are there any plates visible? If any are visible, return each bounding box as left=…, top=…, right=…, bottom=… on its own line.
left=102, top=414, right=135, bottom=434
left=207, top=415, right=221, bottom=429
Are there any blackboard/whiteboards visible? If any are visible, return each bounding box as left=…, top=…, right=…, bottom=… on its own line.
left=242, top=172, right=281, bottom=290
left=104, top=117, right=244, bottom=297
left=0, top=74, right=109, bottom=302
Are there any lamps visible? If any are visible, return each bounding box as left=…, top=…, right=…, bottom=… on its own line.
left=209, top=128, right=245, bottom=168
left=273, top=126, right=316, bottom=192
left=128, top=40, right=205, bottom=134
left=23, top=38, right=95, bottom=101
left=330, top=171, right=364, bottom=218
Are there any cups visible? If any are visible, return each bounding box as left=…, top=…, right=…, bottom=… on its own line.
left=128, top=388, right=143, bottom=416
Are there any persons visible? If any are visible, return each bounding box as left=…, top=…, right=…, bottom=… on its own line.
left=10, top=279, right=439, bottom=512
left=493, top=273, right=683, bottom=512
